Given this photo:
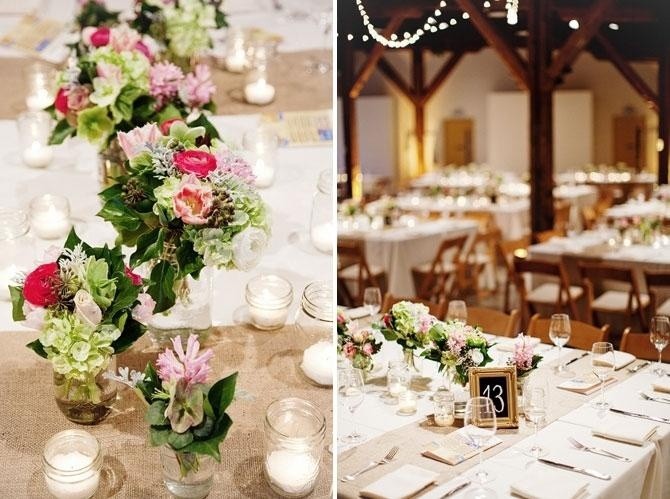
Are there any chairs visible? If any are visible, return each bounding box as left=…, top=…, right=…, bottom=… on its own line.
left=527, top=311, right=609, bottom=352
left=411, top=233, right=468, bottom=303
left=460, top=231, right=494, bottom=301
left=337, top=235, right=386, bottom=309
left=620, top=328, right=670, bottom=361
left=464, top=307, right=520, bottom=337
left=516, top=252, right=581, bottom=327
left=382, top=292, right=443, bottom=322
left=575, top=259, right=649, bottom=330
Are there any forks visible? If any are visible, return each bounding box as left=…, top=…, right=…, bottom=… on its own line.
left=340, top=446, right=400, bottom=483
left=638, top=391, right=669, bottom=404
left=573, top=433, right=631, bottom=463
left=626, top=362, right=648, bottom=374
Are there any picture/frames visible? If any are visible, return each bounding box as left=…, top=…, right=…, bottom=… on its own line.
left=468, top=366, right=519, bottom=428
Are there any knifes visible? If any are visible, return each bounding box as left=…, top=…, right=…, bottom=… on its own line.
left=441, top=482, right=469, bottom=499
left=566, top=352, right=587, bottom=366
left=538, top=458, right=612, bottom=481
left=609, top=408, right=670, bottom=425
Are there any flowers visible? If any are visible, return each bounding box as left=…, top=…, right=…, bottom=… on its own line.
left=419, top=317, right=493, bottom=387
left=506, top=331, right=543, bottom=378
left=103, top=334, right=240, bottom=477
left=337, top=308, right=383, bottom=372
left=45, top=0, right=227, bottom=145
left=7, top=226, right=157, bottom=407
left=371, top=298, right=438, bottom=364
left=97, top=116, right=270, bottom=318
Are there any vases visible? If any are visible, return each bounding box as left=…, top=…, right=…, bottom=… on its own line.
left=402, top=349, right=419, bottom=374
left=160, top=443, right=214, bottom=499
left=52, top=351, right=115, bottom=424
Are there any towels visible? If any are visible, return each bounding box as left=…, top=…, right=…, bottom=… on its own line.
left=497, top=332, right=540, bottom=352
left=591, top=412, right=659, bottom=447
left=595, top=349, right=635, bottom=370
left=652, top=376, right=670, bottom=393
left=359, top=463, right=439, bottom=499
left=511, top=462, right=590, bottom=499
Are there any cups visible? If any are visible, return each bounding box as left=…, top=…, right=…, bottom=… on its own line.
left=309, top=167, right=335, bottom=256
left=549, top=313, right=572, bottom=377
left=522, top=375, right=553, bottom=458
left=295, top=281, right=333, bottom=351
left=338, top=369, right=366, bottom=444
left=363, top=287, right=383, bottom=335
left=397, top=390, right=417, bottom=414
left=241, top=39, right=278, bottom=104
left=262, top=398, right=326, bottom=498
left=463, top=397, right=496, bottom=483
left=17, top=110, right=52, bottom=168
left=244, top=274, right=294, bottom=332
left=239, top=128, right=280, bottom=190
left=41, top=429, right=102, bottom=499
left=27, top=192, right=72, bottom=242
left=387, top=359, right=411, bottom=398
left=433, top=391, right=455, bottom=426
left=23, top=62, right=56, bottom=107
left=591, top=341, right=616, bottom=411
left=445, top=300, right=466, bottom=328
left=649, top=316, right=669, bottom=376
